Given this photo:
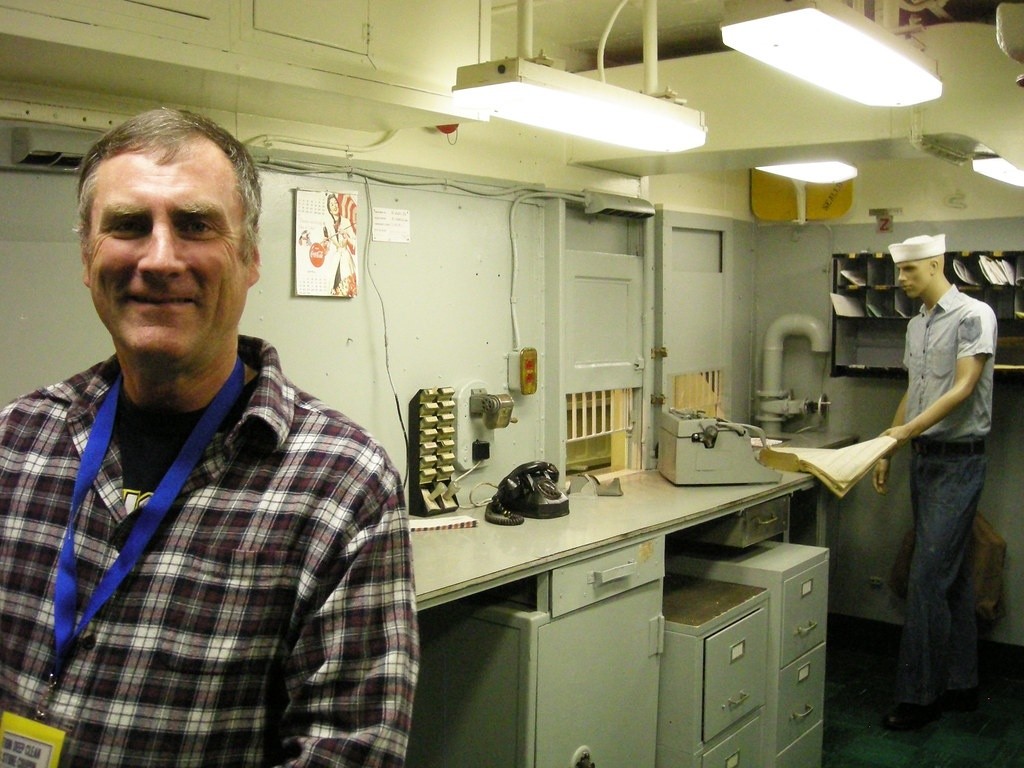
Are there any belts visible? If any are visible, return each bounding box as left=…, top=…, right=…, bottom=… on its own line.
left=911, top=438, right=985, bottom=455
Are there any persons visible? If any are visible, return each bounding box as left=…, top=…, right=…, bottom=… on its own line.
left=0, top=105, right=421, bottom=768
left=322, top=193, right=357, bottom=298
left=875, top=234, right=998, bottom=736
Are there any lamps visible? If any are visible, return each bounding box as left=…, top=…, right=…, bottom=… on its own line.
left=451, top=0, right=708, bottom=153
left=720, top=0, right=943, bottom=108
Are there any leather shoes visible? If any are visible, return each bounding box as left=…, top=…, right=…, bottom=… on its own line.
left=943, top=687, right=977, bottom=713
left=886, top=695, right=943, bottom=731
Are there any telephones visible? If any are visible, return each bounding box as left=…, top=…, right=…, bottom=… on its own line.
left=499, top=460, right=570, bottom=519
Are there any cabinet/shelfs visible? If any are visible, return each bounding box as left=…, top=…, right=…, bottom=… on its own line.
left=830, top=249, right=1024, bottom=380
left=405, top=536, right=830, bottom=768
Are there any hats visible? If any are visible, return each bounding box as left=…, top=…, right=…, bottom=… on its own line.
left=888, top=233, right=946, bottom=264
